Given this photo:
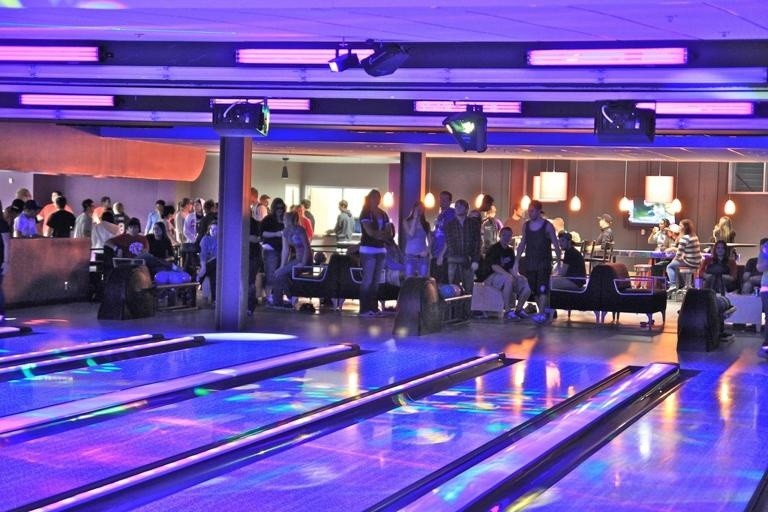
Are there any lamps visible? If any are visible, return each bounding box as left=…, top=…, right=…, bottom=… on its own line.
left=0, top=40, right=114, bottom=63
left=441, top=103, right=489, bottom=153
left=524, top=46, right=700, bottom=68
left=234, top=48, right=376, bottom=71
left=19, top=94, right=123, bottom=109
left=208, top=98, right=320, bottom=113
left=412, top=101, right=529, bottom=116
left=361, top=42, right=412, bottom=77
left=329, top=42, right=362, bottom=73
left=633, top=101, right=758, bottom=118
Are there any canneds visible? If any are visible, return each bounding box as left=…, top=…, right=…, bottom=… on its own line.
left=754, top=287, right=760, bottom=297
left=694, top=277, right=703, bottom=290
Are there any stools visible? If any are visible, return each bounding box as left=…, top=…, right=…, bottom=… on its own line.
left=177, top=242, right=201, bottom=305
left=633, top=264, right=652, bottom=290
left=674, top=266, right=697, bottom=303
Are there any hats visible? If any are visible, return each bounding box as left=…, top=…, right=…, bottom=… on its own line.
left=125, top=215, right=142, bottom=233
left=559, top=233, right=577, bottom=246
left=11, top=198, right=44, bottom=213
left=664, top=224, right=680, bottom=233
left=596, top=213, right=613, bottom=224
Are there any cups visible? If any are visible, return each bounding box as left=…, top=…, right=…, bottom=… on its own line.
left=484, top=224, right=497, bottom=246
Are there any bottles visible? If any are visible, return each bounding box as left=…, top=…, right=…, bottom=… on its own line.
left=694, top=269, right=703, bottom=289
left=12, top=231, right=33, bottom=238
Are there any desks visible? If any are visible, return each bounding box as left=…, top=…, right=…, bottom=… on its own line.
left=593, top=249, right=715, bottom=299
left=724, top=291, right=763, bottom=333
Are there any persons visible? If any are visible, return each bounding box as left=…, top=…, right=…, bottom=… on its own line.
left=648, top=220, right=702, bottom=293
left=248, top=186, right=586, bottom=323
left=586, top=213, right=614, bottom=254
left=2, top=188, right=218, bottom=309
left=698, top=217, right=768, bottom=350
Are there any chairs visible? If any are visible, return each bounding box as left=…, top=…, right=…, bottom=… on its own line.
left=97, top=252, right=116, bottom=302
left=548, top=258, right=613, bottom=324
left=283, top=254, right=347, bottom=311
left=600, top=261, right=667, bottom=329
left=579, top=239, right=596, bottom=274
left=337, top=253, right=400, bottom=312
left=469, top=254, right=516, bottom=320
left=727, top=264, right=746, bottom=295
left=583, top=240, right=616, bottom=279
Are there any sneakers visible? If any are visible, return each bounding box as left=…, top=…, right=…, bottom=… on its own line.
left=246, top=296, right=386, bottom=317
left=504, top=304, right=557, bottom=324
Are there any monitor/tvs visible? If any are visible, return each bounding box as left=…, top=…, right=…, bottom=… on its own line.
left=594, top=107, right=655, bottom=143
left=623, top=195, right=678, bottom=229
left=212, top=104, right=270, bottom=136
